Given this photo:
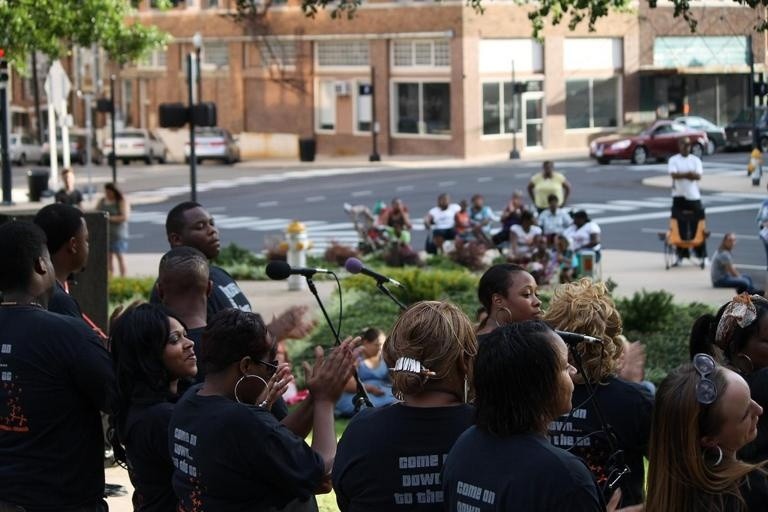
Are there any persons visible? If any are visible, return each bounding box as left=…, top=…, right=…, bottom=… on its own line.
left=666, top=136, right=709, bottom=269
left=466, top=262, right=545, bottom=407
left=153, top=244, right=288, bottom=425
left=109, top=302, right=294, bottom=512
left=28, top=203, right=91, bottom=318
left=439, top=318, right=645, bottom=512
left=709, top=232, right=767, bottom=296
left=538, top=276, right=657, bottom=512
left=688, top=292, right=767, bottom=512
left=647, top=351, right=768, bottom=511
left=271, top=338, right=308, bottom=404
left=755, top=182, right=768, bottom=246
left=54, top=169, right=84, bottom=210
left=331, top=301, right=483, bottom=512
left=168, top=306, right=368, bottom=512
left=1, top=216, right=131, bottom=512
left=163, top=199, right=322, bottom=350
left=371, top=158, right=605, bottom=292
left=95, top=181, right=132, bottom=279
left=333, top=324, right=404, bottom=418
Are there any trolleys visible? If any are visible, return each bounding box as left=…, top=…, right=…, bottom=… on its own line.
left=659, top=204, right=712, bottom=268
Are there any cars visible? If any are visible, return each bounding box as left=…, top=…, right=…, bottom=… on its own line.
left=9, top=132, right=45, bottom=171
left=660, top=114, right=727, bottom=163
left=103, top=128, right=169, bottom=166
left=42, top=130, right=101, bottom=168
left=182, top=125, right=242, bottom=168
left=587, top=119, right=710, bottom=171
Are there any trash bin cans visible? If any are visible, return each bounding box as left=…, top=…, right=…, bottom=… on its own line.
left=299, top=138, right=316, bottom=161
left=27, top=170, right=48, bottom=201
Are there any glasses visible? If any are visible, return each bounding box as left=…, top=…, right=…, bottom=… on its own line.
left=693, top=353, right=718, bottom=404
left=255, top=359, right=278, bottom=373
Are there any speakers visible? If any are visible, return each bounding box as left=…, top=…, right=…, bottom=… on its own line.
left=0, top=209, right=109, bottom=334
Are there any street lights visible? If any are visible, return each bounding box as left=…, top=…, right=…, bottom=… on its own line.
left=189, top=33, right=204, bottom=128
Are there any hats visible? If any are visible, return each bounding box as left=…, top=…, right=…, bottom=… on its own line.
left=568, top=210, right=590, bottom=223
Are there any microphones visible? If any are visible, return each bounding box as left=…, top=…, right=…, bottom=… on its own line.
left=345, top=258, right=401, bottom=289
left=266, top=260, right=328, bottom=281
left=554, top=328, right=601, bottom=346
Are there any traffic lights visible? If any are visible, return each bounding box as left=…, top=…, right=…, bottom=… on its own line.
left=0, top=48, right=10, bottom=90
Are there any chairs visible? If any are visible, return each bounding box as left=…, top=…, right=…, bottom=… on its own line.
left=658, top=209, right=711, bottom=268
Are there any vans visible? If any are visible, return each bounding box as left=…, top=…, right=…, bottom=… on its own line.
left=724, top=105, right=767, bottom=154
